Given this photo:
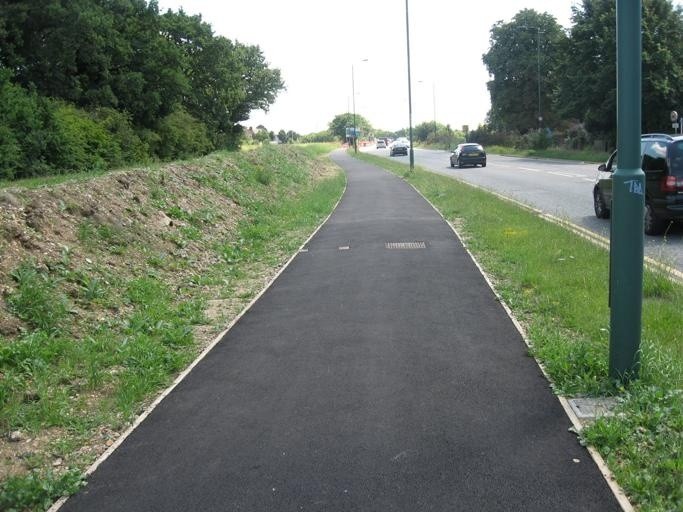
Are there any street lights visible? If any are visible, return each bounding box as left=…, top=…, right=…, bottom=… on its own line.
left=351, top=59, right=368, bottom=151
left=517, top=25, right=542, bottom=131
left=418, top=79, right=437, bottom=143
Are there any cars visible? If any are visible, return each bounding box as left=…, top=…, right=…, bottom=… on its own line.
left=375, top=137, right=409, bottom=157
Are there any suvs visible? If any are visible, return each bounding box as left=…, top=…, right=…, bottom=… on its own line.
left=592, top=132, right=683, bottom=235
left=449, top=142, right=486, bottom=169
